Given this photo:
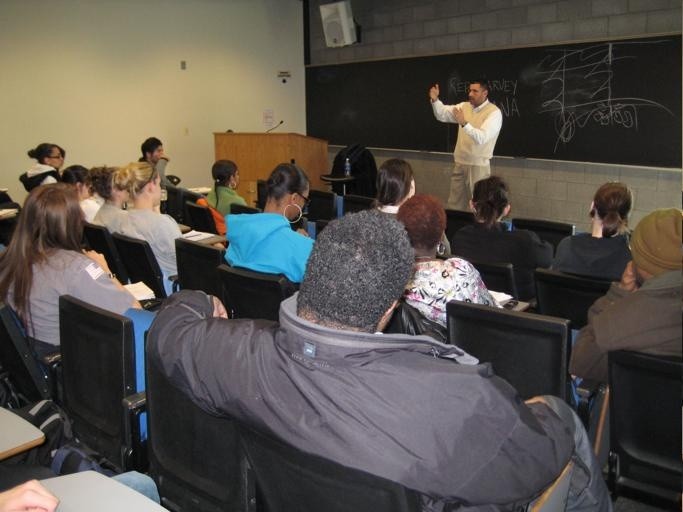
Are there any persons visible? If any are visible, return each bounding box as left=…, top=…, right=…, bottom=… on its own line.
left=451, top=177, right=548, bottom=305
left=0, top=184, right=142, bottom=355
left=205, top=160, right=244, bottom=218
left=61, top=165, right=87, bottom=205
left=114, top=162, right=183, bottom=296
left=557, top=181, right=632, bottom=282
left=570, top=207, right=683, bottom=374
left=429, top=78, right=503, bottom=212
left=144, top=210, right=614, bottom=512
left=0, top=478, right=60, bottom=512
left=396, top=194, right=494, bottom=329
left=88, top=166, right=128, bottom=233
left=224, top=163, right=316, bottom=283
left=370, top=159, right=416, bottom=214
left=19, top=143, right=64, bottom=192
left=137, top=138, right=174, bottom=189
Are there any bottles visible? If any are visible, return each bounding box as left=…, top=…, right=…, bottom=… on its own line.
left=344, top=158, right=351, bottom=177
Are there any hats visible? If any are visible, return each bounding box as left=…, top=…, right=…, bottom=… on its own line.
left=629, top=208, right=683, bottom=274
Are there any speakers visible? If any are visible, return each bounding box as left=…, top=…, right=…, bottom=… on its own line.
left=319, top=2, right=356, bottom=47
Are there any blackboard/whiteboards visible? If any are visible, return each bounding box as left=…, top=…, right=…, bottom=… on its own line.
left=305, top=30, right=682, bottom=174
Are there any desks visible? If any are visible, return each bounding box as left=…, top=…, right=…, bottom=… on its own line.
left=213, top=132, right=330, bottom=210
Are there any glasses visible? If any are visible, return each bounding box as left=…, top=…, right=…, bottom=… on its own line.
left=297, top=192, right=312, bottom=208
left=47, top=153, right=62, bottom=160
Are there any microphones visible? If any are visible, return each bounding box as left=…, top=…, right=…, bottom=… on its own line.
left=266, top=120, right=284, bottom=132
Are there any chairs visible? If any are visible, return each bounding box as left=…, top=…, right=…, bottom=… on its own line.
left=59, top=294, right=146, bottom=477
left=144, top=330, right=256, bottom=512
left=183, top=200, right=227, bottom=237
left=0, top=303, right=60, bottom=412
left=469, top=258, right=538, bottom=314
left=342, top=194, right=378, bottom=216
left=530, top=267, right=643, bottom=327
left=230, top=204, right=262, bottom=215
left=512, top=218, right=576, bottom=261
left=112, top=231, right=179, bottom=299
left=83, top=221, right=128, bottom=285
left=167, top=186, right=184, bottom=224
left=175, top=237, right=225, bottom=306
left=256, top=179, right=270, bottom=212
left=607, top=348, right=683, bottom=512
left=235, top=420, right=422, bottom=512
left=384, top=299, right=448, bottom=345
left=216, top=264, right=300, bottom=323
left=302, top=189, right=337, bottom=223
left=446, top=300, right=601, bottom=419
left=443, top=207, right=475, bottom=245
